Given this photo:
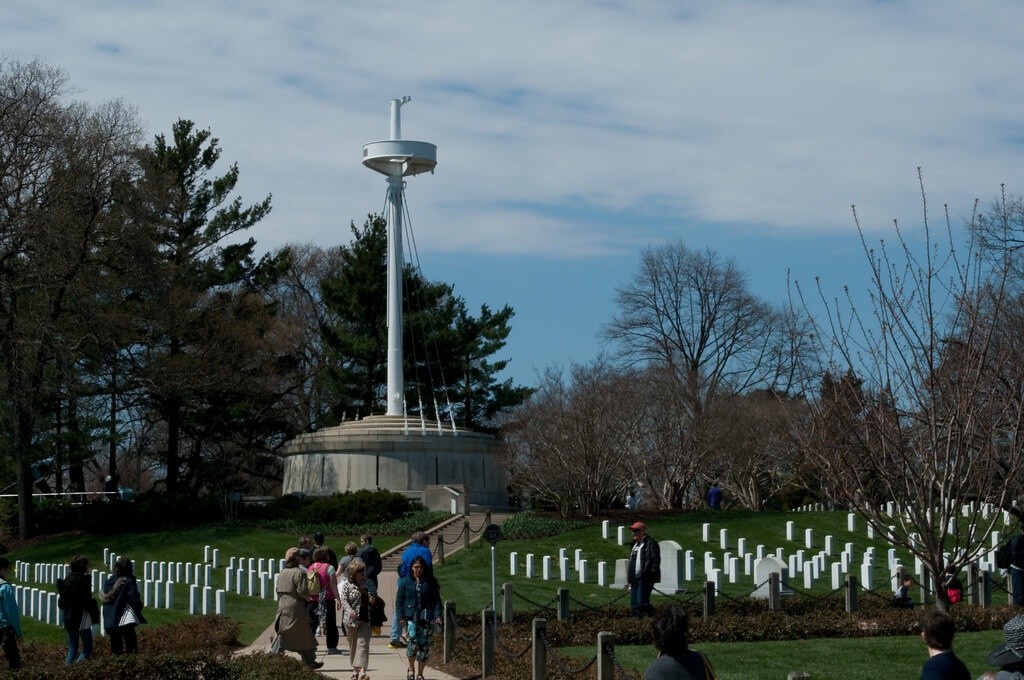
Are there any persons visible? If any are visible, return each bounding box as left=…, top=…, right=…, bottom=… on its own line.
left=919, top=611, right=971, bottom=680
left=56, top=555, right=100, bottom=664
left=893, top=575, right=914, bottom=610
left=946, top=565, right=963, bottom=604
left=0, top=557, right=23, bottom=670
left=387, top=531, right=433, bottom=647
left=271, top=547, right=324, bottom=670
left=99, top=557, right=149, bottom=659
left=642, top=605, right=715, bottom=680
left=341, top=562, right=378, bottom=680
left=396, top=556, right=442, bottom=680
left=300, top=532, right=343, bottom=655
left=1010, top=525, right=1024, bottom=608
left=336, top=533, right=382, bottom=590
left=708, top=481, right=723, bottom=511
left=977, top=613, right=1024, bottom=680
left=624, top=489, right=635, bottom=510
left=628, top=521, right=661, bottom=618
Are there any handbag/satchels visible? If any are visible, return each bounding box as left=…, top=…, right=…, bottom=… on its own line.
left=359, top=589, right=387, bottom=627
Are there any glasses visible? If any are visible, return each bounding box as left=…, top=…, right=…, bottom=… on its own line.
left=356, top=568, right=364, bottom=573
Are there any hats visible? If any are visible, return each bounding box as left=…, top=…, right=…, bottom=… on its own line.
left=285, top=547, right=300, bottom=564
left=629, top=522, right=645, bottom=530
left=988, top=613, right=1024, bottom=664
left=314, top=532, right=325, bottom=542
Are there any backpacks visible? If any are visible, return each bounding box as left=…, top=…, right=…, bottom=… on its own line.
left=307, top=562, right=330, bottom=596
left=996, top=536, right=1020, bottom=569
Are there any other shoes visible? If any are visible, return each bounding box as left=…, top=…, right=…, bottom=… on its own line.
left=328, top=648, right=341, bottom=655
left=407, top=668, right=414, bottom=680
left=360, top=675, right=366, bottom=680
left=351, top=674, right=358, bottom=680
left=389, top=641, right=407, bottom=648
left=418, top=674, right=424, bottom=680
left=312, top=662, right=322, bottom=669
